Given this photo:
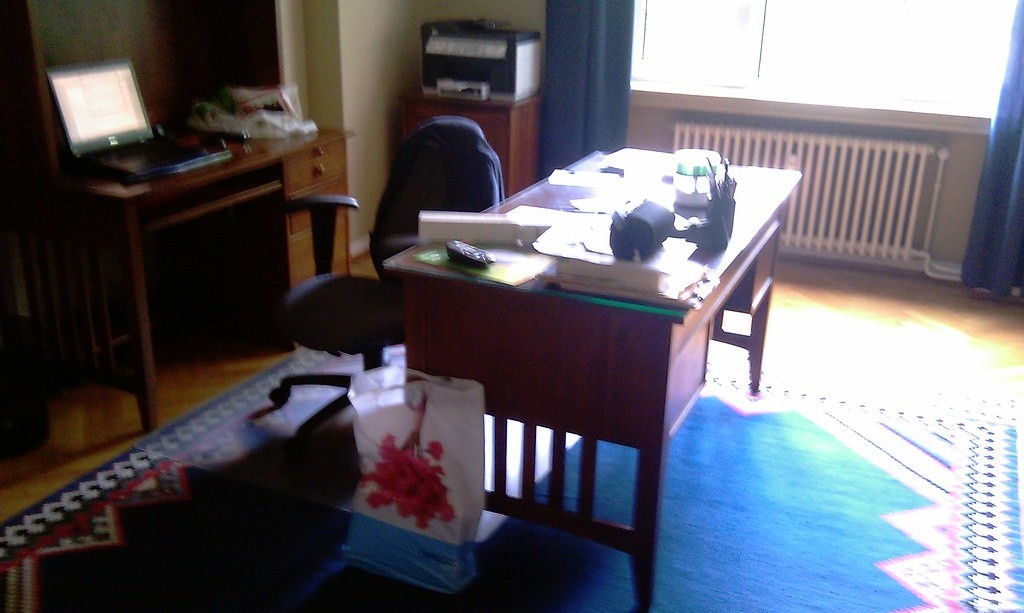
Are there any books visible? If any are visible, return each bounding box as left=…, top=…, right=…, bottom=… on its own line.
left=532, top=215, right=721, bottom=310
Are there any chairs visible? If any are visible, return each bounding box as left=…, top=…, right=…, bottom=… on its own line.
left=265, top=116, right=506, bottom=458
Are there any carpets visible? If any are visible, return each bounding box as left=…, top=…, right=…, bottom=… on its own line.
left=0, top=348, right=1024, bottom=613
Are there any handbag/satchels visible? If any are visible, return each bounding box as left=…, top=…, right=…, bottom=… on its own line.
left=341, top=364, right=484, bottom=594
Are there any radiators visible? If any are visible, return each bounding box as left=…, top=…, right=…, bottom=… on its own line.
left=667, top=115, right=936, bottom=262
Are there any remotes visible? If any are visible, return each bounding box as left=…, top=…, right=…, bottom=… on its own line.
left=446, top=239, right=495, bottom=266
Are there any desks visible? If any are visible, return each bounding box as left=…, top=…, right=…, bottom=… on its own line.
left=382, top=148, right=802, bottom=613
left=54, top=125, right=349, bottom=432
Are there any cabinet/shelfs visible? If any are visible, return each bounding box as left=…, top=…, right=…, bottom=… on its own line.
left=397, top=89, right=545, bottom=198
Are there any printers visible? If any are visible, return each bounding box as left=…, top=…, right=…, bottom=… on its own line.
left=420, top=18, right=543, bottom=102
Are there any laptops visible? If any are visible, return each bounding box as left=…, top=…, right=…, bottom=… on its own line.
left=47, top=56, right=232, bottom=183
